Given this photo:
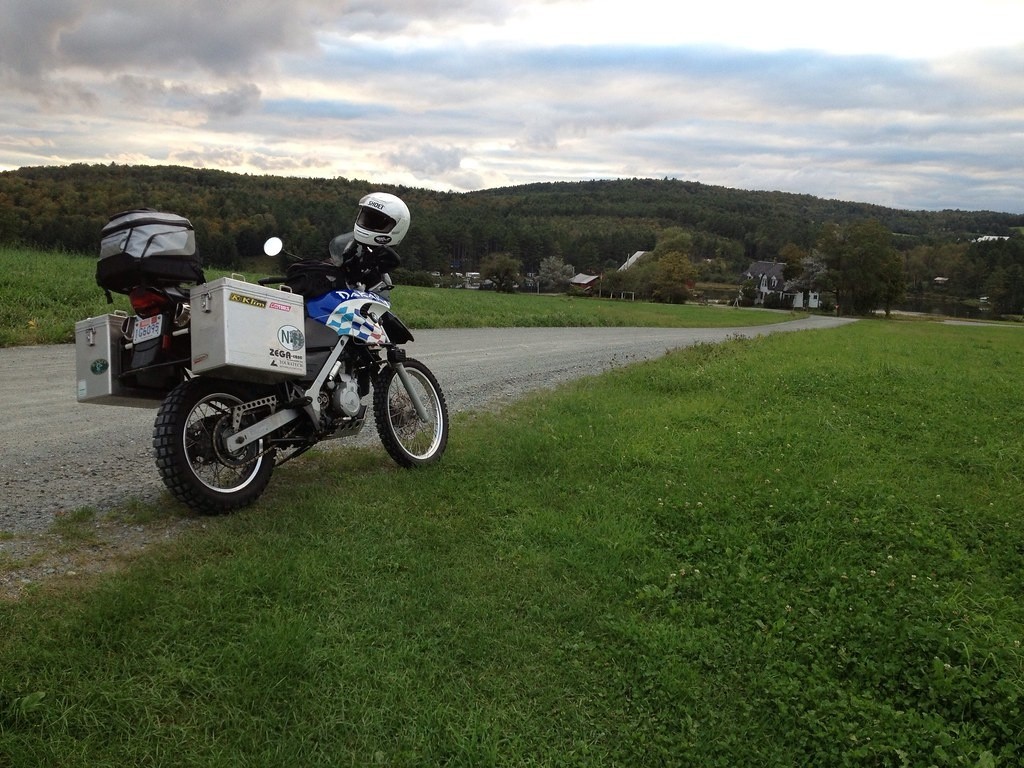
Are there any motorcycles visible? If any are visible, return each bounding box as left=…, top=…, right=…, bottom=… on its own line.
left=72, top=235, right=449, bottom=515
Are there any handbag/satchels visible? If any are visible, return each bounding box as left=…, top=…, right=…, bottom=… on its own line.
left=96, top=207, right=202, bottom=290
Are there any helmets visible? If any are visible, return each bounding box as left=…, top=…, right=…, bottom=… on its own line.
left=353, top=192, right=410, bottom=247
